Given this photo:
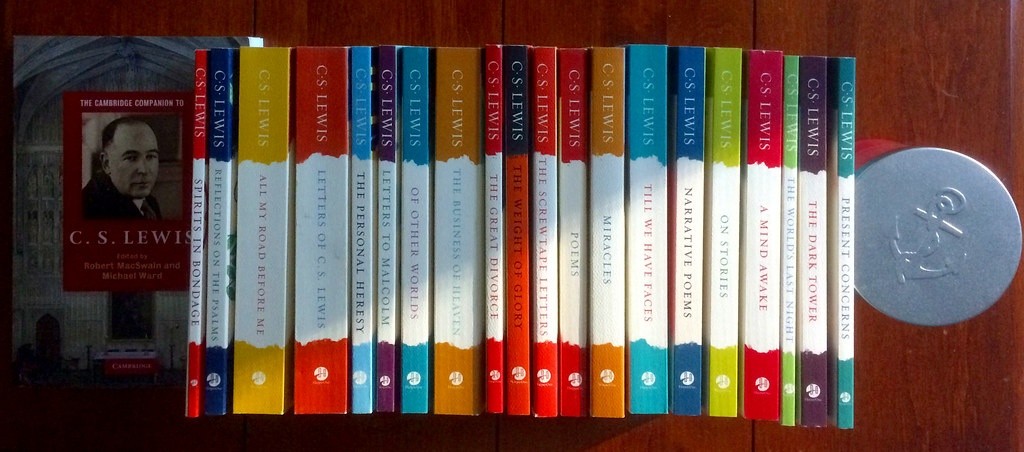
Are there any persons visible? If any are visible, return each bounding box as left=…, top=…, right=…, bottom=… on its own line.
left=82, top=116, right=163, bottom=221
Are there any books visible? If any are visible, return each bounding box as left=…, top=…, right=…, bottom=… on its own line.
left=187, top=41, right=856, bottom=430
